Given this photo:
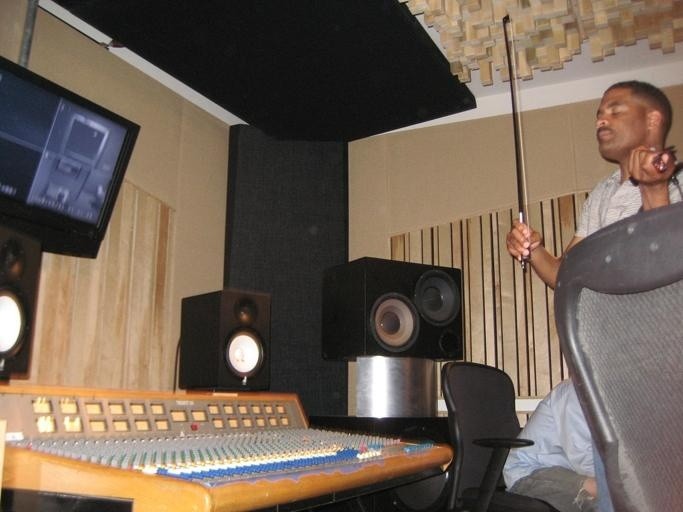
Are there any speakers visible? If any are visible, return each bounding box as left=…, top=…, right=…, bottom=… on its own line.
left=178, top=288, right=273, bottom=393
left=221, top=124, right=349, bottom=417
left=0, top=228, right=43, bottom=383
left=320, top=256, right=463, bottom=361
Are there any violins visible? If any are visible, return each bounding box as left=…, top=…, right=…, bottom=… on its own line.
left=628, top=145, right=679, bottom=187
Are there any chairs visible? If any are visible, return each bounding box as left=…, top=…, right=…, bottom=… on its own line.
left=438, top=361, right=559, bottom=511
left=552, top=203, right=683, bottom=511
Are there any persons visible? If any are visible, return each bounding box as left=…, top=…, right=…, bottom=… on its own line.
left=502, top=79, right=683, bottom=512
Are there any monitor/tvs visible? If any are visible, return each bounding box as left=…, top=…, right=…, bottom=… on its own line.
left=0, top=53, right=141, bottom=261
